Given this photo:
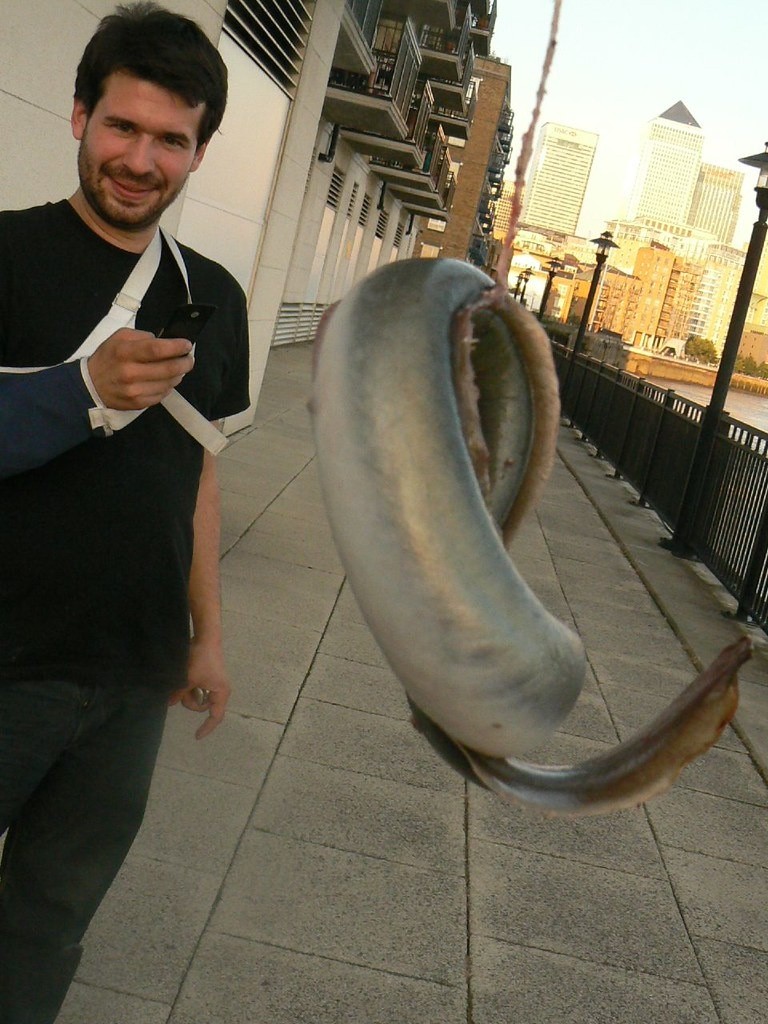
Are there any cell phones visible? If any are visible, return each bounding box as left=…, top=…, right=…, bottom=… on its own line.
left=156, top=304, right=217, bottom=356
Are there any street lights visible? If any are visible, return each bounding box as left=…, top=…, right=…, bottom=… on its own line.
left=659, top=143, right=768, bottom=567
left=514, top=257, right=564, bottom=319
left=561, top=232, right=621, bottom=414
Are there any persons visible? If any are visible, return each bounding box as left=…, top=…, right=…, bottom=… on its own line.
left=1, top=3, right=253, bottom=1020
